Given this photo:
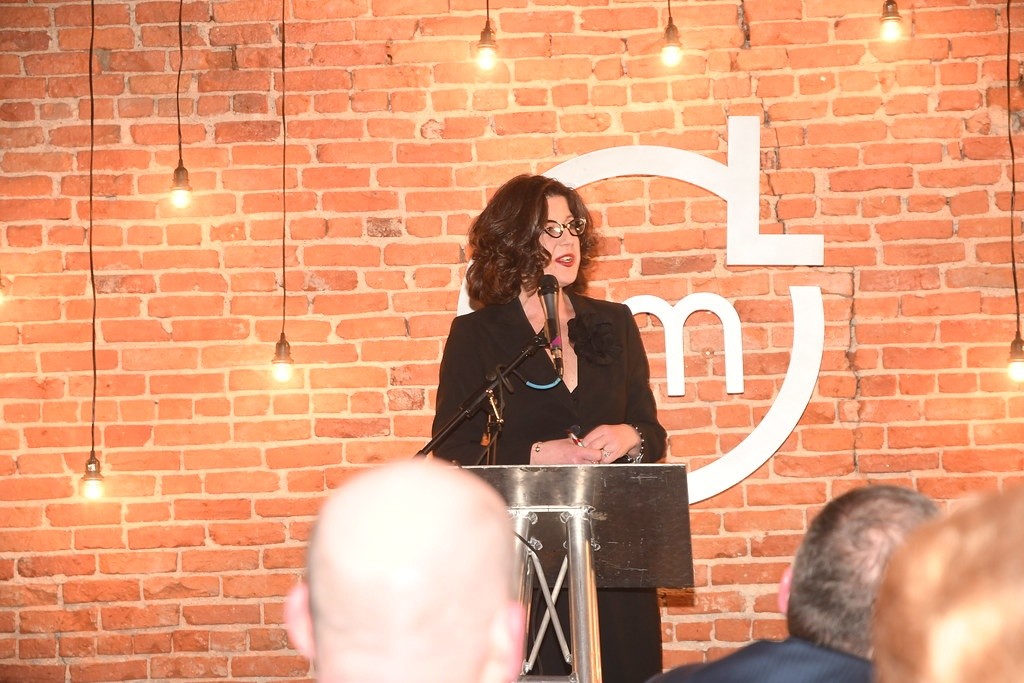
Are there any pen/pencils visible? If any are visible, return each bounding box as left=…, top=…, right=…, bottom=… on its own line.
left=566, top=428, right=600, bottom=465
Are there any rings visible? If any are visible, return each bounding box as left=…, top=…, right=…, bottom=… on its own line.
left=601, top=448, right=612, bottom=456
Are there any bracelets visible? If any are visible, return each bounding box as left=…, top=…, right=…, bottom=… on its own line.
left=535, top=441, right=543, bottom=451
left=623, top=422, right=645, bottom=462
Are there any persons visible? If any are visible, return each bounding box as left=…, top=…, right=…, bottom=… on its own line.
left=431, top=173, right=669, bottom=683
left=872, top=484, right=1024, bottom=683
left=645, top=483, right=940, bottom=683
left=284, top=457, right=528, bottom=683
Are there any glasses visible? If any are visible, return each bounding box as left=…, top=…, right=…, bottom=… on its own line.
left=544, top=216, right=586, bottom=238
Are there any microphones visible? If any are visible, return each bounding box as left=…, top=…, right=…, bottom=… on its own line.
left=537, top=274, right=564, bottom=378
